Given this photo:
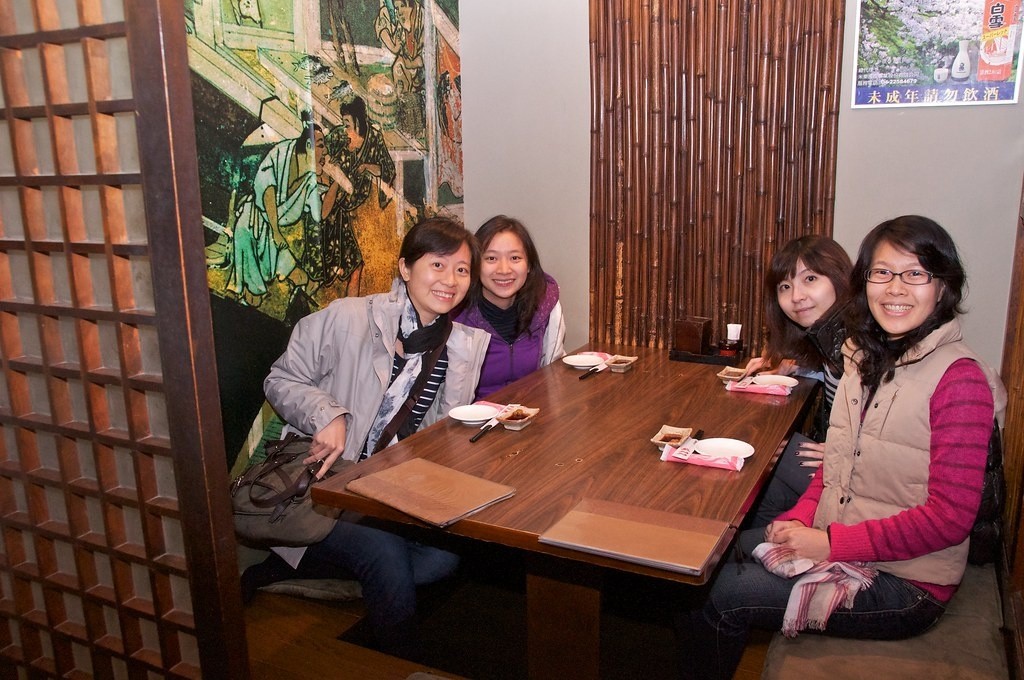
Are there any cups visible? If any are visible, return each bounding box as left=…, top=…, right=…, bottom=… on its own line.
left=934, top=69, right=948, bottom=83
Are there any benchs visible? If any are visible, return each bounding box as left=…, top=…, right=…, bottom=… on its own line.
left=731, top=531, right=1013, bottom=680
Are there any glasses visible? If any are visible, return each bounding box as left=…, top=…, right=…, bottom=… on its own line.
left=863, top=268, right=935, bottom=285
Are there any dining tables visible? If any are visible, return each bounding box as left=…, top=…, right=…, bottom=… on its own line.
left=310, top=343, right=822, bottom=680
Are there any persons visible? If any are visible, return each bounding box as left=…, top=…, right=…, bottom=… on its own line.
left=264, top=216, right=491, bottom=634
left=670, top=214, right=1007, bottom=680
left=449, top=215, right=566, bottom=404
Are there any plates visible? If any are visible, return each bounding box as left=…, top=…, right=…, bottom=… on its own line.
left=694, top=437, right=755, bottom=461
left=752, top=375, right=799, bottom=388
left=562, top=355, right=604, bottom=369
left=448, top=405, right=499, bottom=425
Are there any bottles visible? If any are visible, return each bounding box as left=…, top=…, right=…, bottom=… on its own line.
left=951, top=40, right=970, bottom=81
left=718, top=338, right=743, bottom=357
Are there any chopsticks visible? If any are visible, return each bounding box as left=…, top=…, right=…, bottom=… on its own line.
left=693, top=429, right=704, bottom=439
left=750, top=371, right=758, bottom=376
left=469, top=403, right=521, bottom=442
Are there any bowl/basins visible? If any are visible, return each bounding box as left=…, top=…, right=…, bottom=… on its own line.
left=497, top=405, right=541, bottom=430
left=716, top=366, right=749, bottom=385
left=650, top=424, right=692, bottom=452
left=604, top=354, right=639, bottom=373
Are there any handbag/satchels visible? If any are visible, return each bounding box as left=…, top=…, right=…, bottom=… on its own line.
left=229, top=433, right=356, bottom=545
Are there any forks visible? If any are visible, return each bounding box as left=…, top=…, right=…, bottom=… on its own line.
left=579, top=367, right=599, bottom=380
